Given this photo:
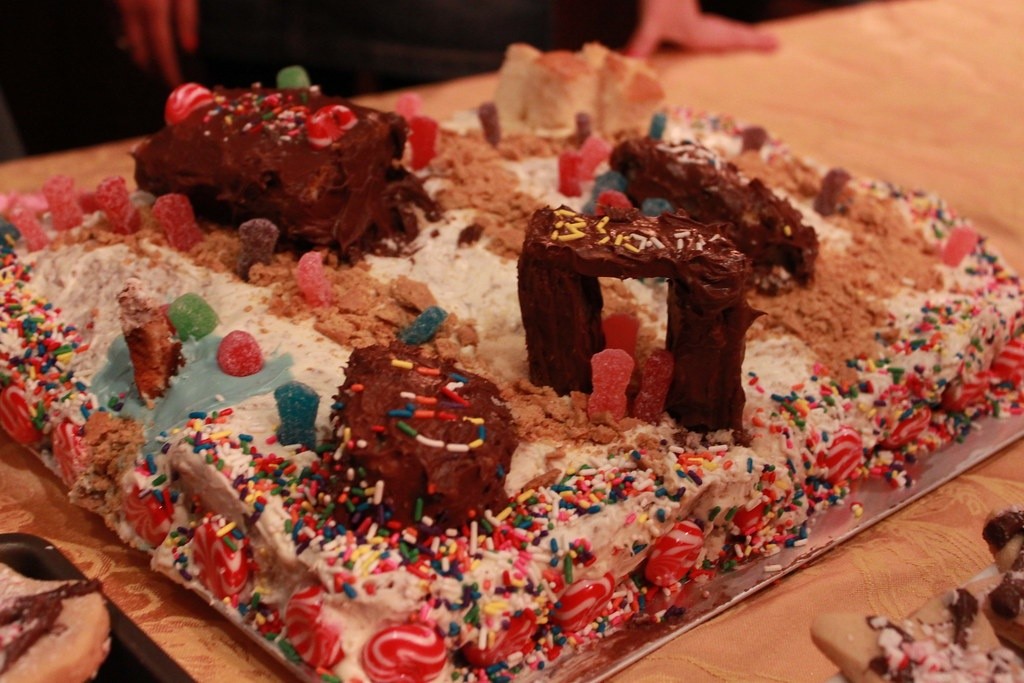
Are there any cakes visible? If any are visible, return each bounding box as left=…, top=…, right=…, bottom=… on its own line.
left=809, top=505, right=1024, bottom=683
left=0, top=41, right=1024, bottom=683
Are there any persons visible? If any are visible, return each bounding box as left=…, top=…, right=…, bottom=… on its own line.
left=0, top=0, right=781, bottom=161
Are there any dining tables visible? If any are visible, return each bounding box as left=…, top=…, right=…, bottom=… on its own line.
left=0, top=0, right=1024, bottom=683
